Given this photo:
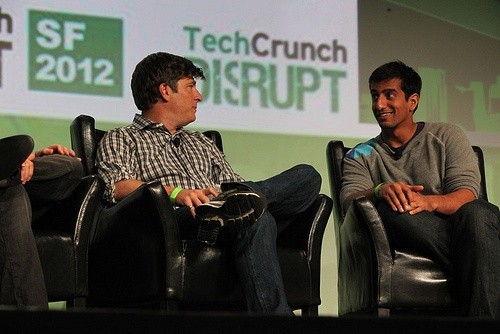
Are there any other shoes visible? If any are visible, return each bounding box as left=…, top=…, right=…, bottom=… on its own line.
left=0, top=134, right=35, bottom=182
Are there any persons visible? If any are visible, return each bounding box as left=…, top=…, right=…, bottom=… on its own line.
left=95, top=52, right=322, bottom=316
left=0, top=134, right=84, bottom=310
left=340, top=60, right=500, bottom=316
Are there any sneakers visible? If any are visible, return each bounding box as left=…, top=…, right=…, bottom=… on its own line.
left=194, top=190, right=268, bottom=231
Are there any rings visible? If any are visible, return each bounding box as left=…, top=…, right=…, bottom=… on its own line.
left=188, top=205, right=193, bottom=209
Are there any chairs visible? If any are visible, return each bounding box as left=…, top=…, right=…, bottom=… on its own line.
left=326, top=141, right=487, bottom=318
left=24, top=174, right=106, bottom=312
left=70, top=115, right=333, bottom=318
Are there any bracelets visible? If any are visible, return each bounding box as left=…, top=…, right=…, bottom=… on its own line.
left=170, top=187, right=184, bottom=207
left=375, top=183, right=384, bottom=198
